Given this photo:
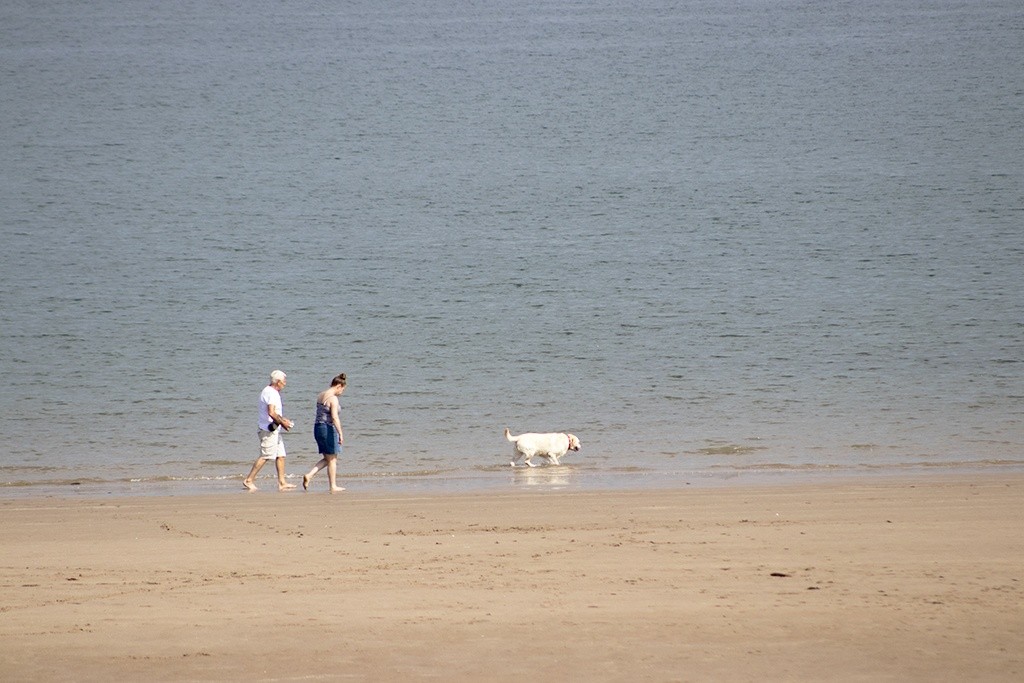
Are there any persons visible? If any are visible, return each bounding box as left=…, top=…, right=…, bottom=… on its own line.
left=302, top=372, right=348, bottom=493
left=242, top=368, right=298, bottom=491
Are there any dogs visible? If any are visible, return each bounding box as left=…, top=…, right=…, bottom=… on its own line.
left=505, top=426, right=581, bottom=468
